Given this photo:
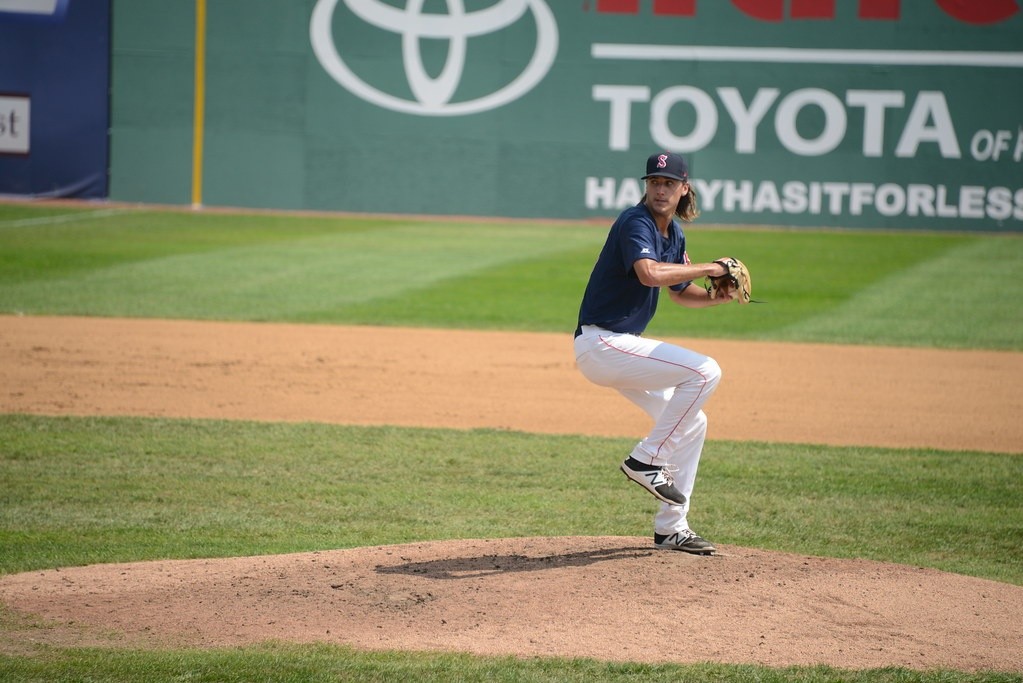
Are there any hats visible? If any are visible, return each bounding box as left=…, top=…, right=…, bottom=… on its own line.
left=640, top=151, right=688, bottom=181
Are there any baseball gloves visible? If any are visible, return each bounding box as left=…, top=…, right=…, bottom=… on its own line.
left=706, top=256, right=752, bottom=304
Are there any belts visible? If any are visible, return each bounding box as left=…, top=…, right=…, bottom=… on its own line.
left=573, top=327, right=583, bottom=339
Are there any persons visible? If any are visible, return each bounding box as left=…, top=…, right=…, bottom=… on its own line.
left=574, top=150, right=751, bottom=555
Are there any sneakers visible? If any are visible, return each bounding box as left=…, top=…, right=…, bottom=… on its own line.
left=654, top=529, right=717, bottom=555
left=621, top=455, right=687, bottom=506
